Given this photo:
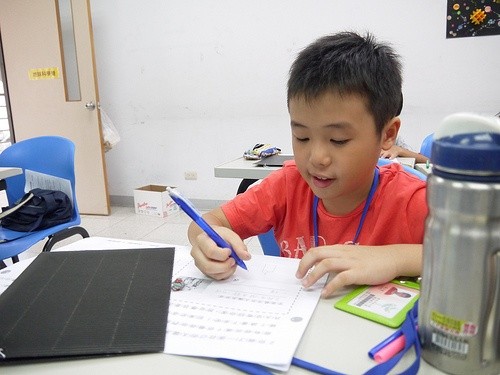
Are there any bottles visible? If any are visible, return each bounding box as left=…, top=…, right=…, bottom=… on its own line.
left=416, top=112, right=500, bottom=375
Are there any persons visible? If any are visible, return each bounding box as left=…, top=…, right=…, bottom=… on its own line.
left=188, top=32, right=428, bottom=296
left=378, top=139, right=428, bottom=164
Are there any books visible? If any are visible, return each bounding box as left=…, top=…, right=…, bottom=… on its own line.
left=45, top=238, right=331, bottom=371
left=24, top=169, right=74, bottom=210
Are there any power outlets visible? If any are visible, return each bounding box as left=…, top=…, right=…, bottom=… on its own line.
left=185, top=172, right=197, bottom=179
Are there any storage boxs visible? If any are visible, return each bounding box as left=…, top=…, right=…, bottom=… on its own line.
left=133, top=184, right=180, bottom=219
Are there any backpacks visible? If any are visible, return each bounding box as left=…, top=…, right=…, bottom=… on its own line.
left=0, top=188, right=72, bottom=232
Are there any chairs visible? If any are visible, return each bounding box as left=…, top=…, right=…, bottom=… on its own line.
left=0, top=135, right=91, bottom=272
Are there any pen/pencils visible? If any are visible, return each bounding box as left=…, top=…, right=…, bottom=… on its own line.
left=368, top=319, right=417, bottom=364
left=165, top=185, right=249, bottom=271
left=426, top=159, right=430, bottom=168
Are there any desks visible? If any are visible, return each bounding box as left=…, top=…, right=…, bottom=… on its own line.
left=215, top=153, right=432, bottom=197
left=0, top=167, right=22, bottom=180
left=0, top=236, right=447, bottom=375
